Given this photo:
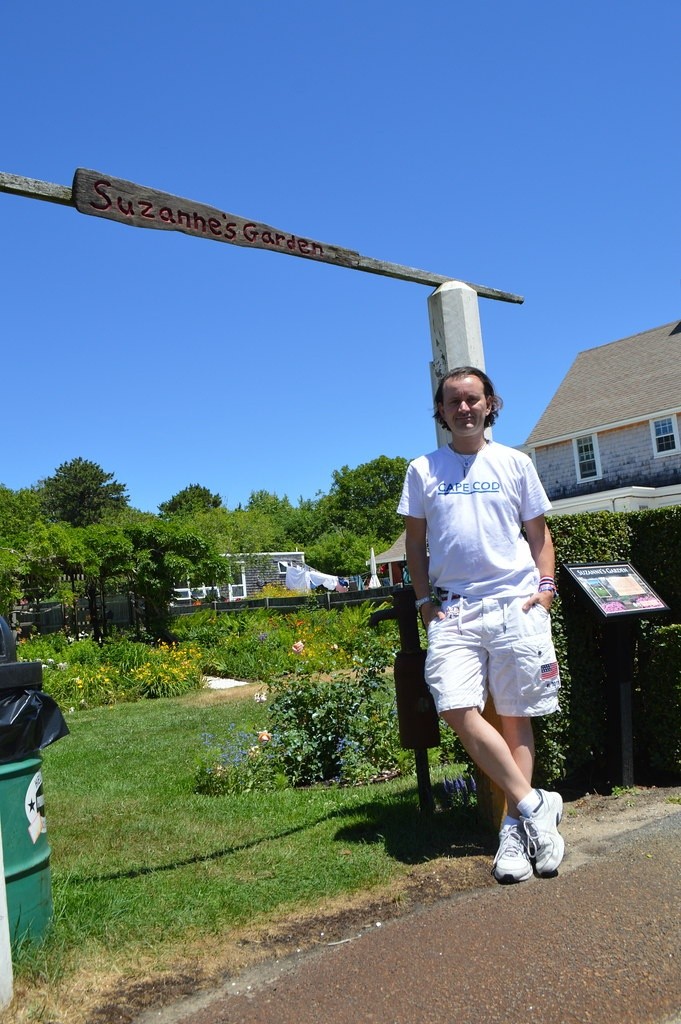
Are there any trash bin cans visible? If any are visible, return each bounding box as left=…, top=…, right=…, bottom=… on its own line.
left=0, top=614, right=51, bottom=948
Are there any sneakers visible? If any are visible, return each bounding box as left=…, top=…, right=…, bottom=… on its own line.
left=493, top=825, right=533, bottom=884
left=519, top=789, right=565, bottom=876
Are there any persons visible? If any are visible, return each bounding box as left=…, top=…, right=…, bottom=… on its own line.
left=395, top=364, right=565, bottom=884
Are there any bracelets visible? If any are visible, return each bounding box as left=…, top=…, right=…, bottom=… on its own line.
left=538, top=575, right=554, bottom=593
left=415, top=596, right=431, bottom=612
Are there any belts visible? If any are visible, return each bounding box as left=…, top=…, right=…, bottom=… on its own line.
left=441, top=591, right=467, bottom=601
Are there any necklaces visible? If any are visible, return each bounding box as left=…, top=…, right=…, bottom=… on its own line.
left=450, top=441, right=487, bottom=478
